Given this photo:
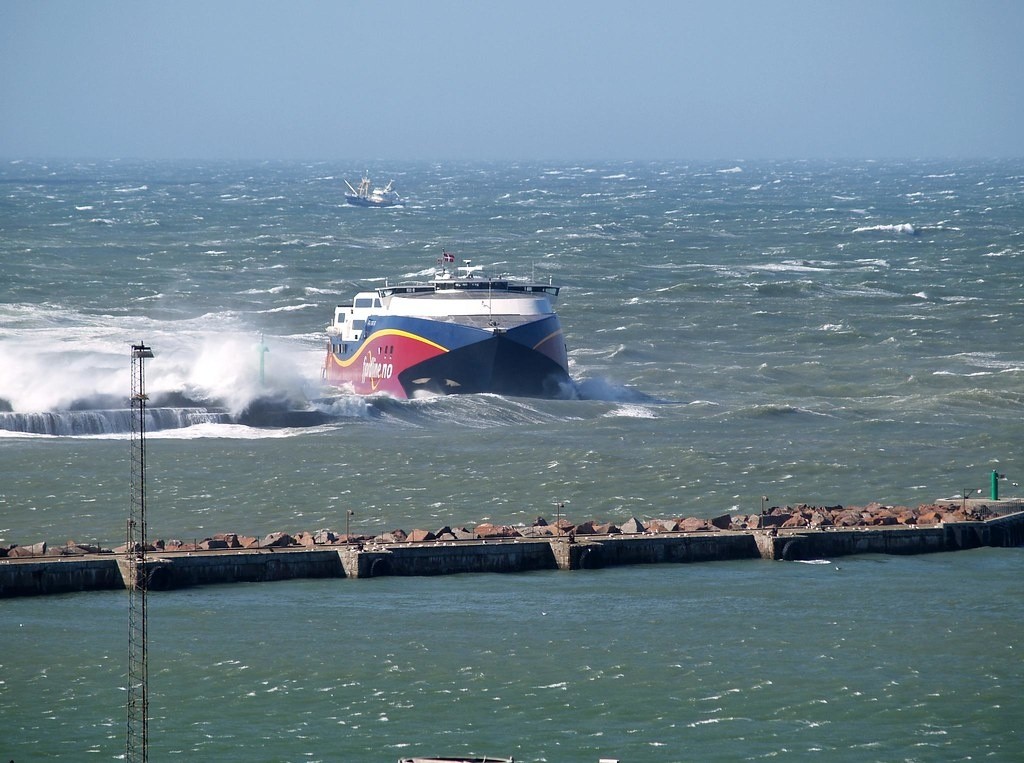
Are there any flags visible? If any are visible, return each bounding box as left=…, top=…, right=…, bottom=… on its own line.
left=444, top=254, right=454, bottom=262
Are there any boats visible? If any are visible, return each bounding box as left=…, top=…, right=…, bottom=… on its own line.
left=317, top=245, right=571, bottom=403
left=342, top=171, right=399, bottom=208
